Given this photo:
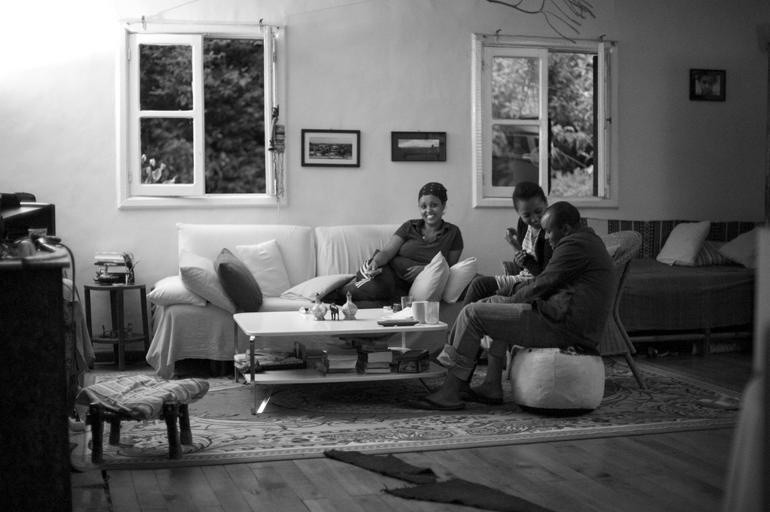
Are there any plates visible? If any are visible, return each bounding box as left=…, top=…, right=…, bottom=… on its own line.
left=377, top=321, right=420, bottom=326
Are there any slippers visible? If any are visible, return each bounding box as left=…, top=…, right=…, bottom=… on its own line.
left=461, top=390, right=507, bottom=405
left=407, top=398, right=465, bottom=411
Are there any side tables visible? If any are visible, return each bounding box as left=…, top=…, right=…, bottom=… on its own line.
left=84, top=282, right=150, bottom=371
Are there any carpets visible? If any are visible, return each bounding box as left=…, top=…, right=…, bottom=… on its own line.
left=71, top=355, right=744, bottom=472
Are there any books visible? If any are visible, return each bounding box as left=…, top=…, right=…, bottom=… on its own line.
left=320, top=338, right=397, bottom=376
left=255, top=350, right=304, bottom=371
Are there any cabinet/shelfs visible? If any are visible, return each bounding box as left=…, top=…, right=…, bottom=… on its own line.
left=0, top=256, right=73, bottom=512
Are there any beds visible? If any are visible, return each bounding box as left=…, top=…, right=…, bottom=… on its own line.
left=619, top=258, right=756, bottom=342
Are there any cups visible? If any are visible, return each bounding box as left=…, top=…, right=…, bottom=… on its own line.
left=401, top=296, right=416, bottom=309
left=425, top=301, right=440, bottom=324
left=411, top=301, right=424, bottom=324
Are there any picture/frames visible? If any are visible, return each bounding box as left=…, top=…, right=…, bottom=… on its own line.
left=689, top=68, right=726, bottom=102
left=301, top=128, right=361, bottom=167
left=391, top=131, right=446, bottom=162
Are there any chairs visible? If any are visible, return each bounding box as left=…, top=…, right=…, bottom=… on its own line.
left=504, top=230, right=649, bottom=389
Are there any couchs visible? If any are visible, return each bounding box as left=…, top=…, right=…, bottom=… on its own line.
left=145, top=222, right=484, bottom=382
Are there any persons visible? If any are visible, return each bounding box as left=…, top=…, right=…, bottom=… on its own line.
left=460, top=182, right=550, bottom=305
left=407, top=202, right=617, bottom=411
left=694, top=71, right=719, bottom=97
left=336, top=183, right=464, bottom=306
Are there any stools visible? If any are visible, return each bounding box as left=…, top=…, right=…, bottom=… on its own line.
left=510, top=347, right=605, bottom=418
left=75, top=373, right=210, bottom=464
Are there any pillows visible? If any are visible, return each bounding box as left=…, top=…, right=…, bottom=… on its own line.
left=408, top=250, right=449, bottom=307
left=280, top=274, right=356, bottom=303
left=146, top=276, right=207, bottom=307
left=442, top=256, right=480, bottom=304
left=717, top=228, right=755, bottom=268
left=213, top=248, right=263, bottom=312
left=356, top=248, right=381, bottom=282
left=694, top=240, right=736, bottom=266
left=607, top=243, right=623, bottom=261
left=180, top=252, right=239, bottom=315
left=236, top=239, right=291, bottom=298
left=656, top=219, right=710, bottom=267
